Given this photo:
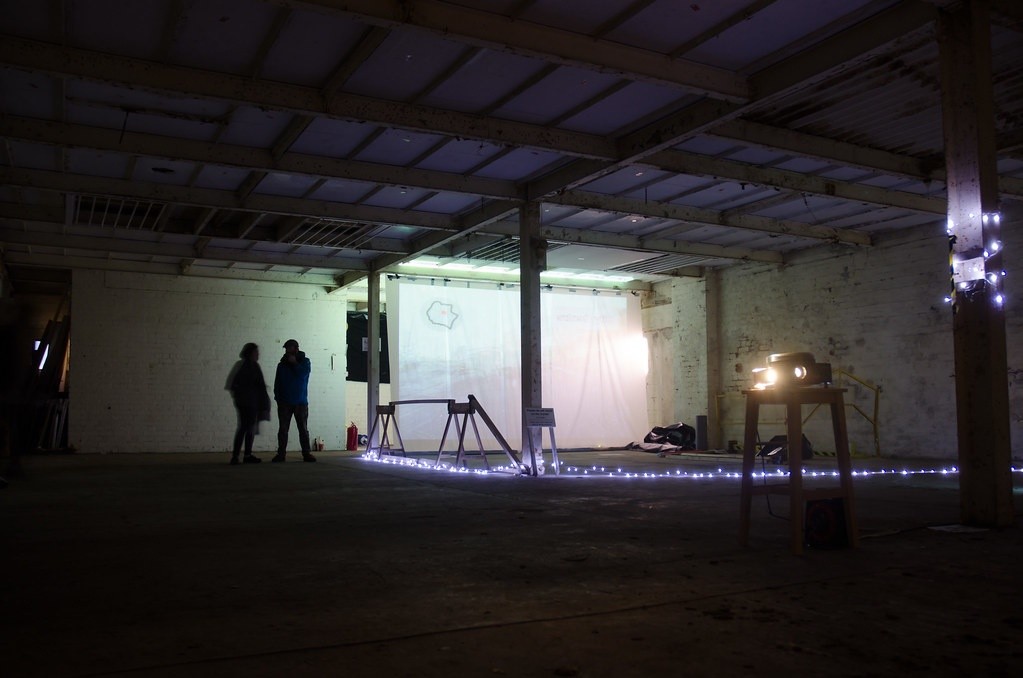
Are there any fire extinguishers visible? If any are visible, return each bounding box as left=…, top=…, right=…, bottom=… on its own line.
left=347, top=421, right=358, bottom=451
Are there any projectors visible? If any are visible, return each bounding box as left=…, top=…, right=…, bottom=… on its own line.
left=752, top=362, right=832, bottom=388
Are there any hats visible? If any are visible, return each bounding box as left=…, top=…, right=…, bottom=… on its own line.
left=283, top=340, right=298, bottom=348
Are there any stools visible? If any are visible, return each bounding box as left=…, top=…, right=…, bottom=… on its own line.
left=742, top=387, right=857, bottom=542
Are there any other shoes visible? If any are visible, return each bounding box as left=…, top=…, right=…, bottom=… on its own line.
left=303, top=454, right=316, bottom=462
left=272, top=454, right=285, bottom=462
left=243, top=455, right=262, bottom=464
left=230, top=456, right=239, bottom=464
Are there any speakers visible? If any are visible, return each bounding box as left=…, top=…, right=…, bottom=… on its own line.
left=803, top=496, right=848, bottom=549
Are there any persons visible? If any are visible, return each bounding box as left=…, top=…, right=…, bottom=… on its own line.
left=272, top=339, right=317, bottom=463
left=224, top=342, right=270, bottom=465
